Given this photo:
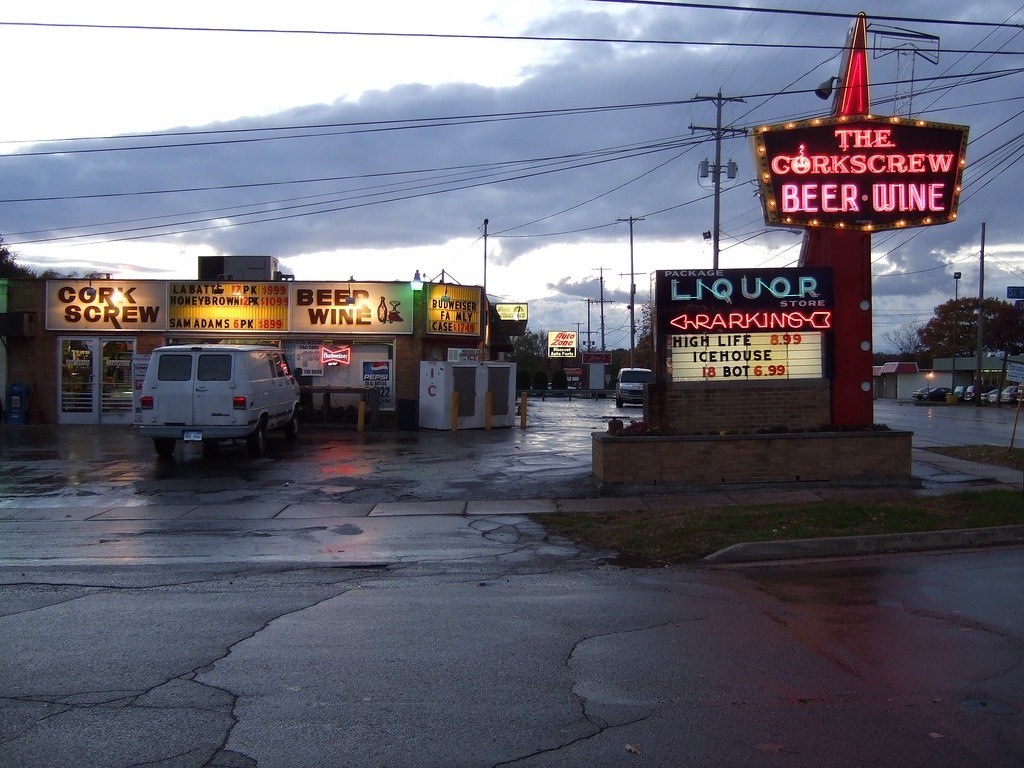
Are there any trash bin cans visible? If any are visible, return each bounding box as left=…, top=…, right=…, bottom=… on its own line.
left=6, top=383, right=29, bottom=425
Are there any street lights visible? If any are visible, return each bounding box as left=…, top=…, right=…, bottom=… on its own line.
left=951, top=271, right=962, bottom=389
left=712, top=228, right=803, bottom=270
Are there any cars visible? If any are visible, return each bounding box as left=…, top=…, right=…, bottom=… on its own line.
left=944, top=384, right=1024, bottom=404
left=922, top=386, right=954, bottom=402
left=911, top=386, right=941, bottom=401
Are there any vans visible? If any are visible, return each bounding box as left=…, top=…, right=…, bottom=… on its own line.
left=613, top=368, right=652, bottom=408
left=133, top=344, right=301, bottom=457
